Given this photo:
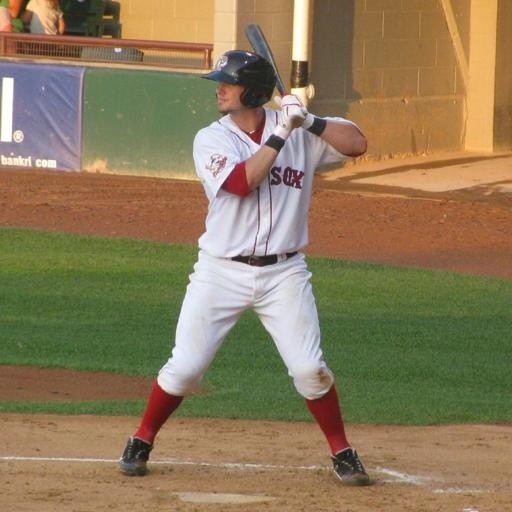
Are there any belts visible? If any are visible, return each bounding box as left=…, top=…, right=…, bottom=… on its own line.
left=229, top=252, right=301, bottom=269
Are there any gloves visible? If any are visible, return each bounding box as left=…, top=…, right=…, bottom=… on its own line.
left=273, top=94, right=314, bottom=129
left=274, top=105, right=306, bottom=141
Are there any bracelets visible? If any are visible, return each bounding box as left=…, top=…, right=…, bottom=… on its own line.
left=264, top=133, right=285, bottom=152
left=306, top=117, right=325, bottom=137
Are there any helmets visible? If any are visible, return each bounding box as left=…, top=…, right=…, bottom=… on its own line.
left=199, top=48, right=277, bottom=108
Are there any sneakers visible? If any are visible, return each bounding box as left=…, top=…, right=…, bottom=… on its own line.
left=328, top=448, right=370, bottom=488
left=120, top=437, right=156, bottom=477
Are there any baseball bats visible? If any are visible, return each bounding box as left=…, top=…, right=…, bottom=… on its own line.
left=245, top=24, right=304, bottom=128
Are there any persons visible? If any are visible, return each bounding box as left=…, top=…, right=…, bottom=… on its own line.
left=23, top=1, right=66, bottom=37
left=1, top=1, right=24, bottom=33
left=118, top=49, right=374, bottom=487
